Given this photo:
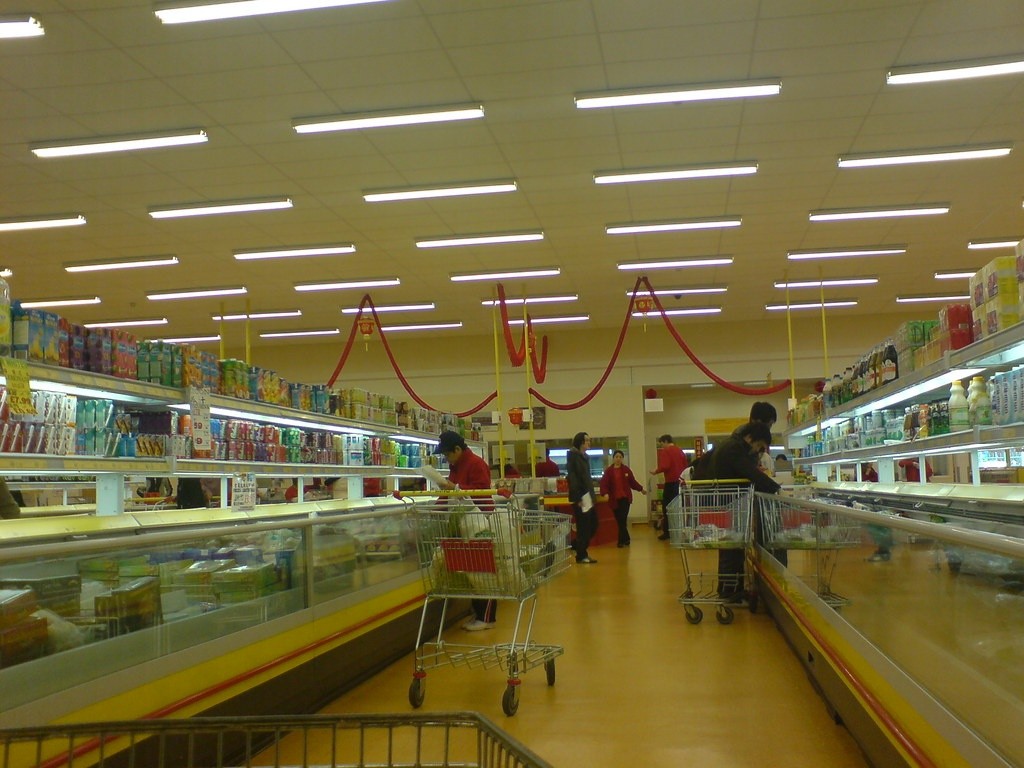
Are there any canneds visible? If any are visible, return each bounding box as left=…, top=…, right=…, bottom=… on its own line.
left=802, top=410, right=903, bottom=457
left=179, top=414, right=444, bottom=468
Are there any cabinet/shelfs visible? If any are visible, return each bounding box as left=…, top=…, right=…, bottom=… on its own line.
left=783, top=320, right=1024, bottom=511
left=0, top=356, right=488, bottom=562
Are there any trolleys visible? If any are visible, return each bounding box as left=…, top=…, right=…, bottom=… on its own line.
left=758, top=495, right=861, bottom=611
left=665, top=478, right=756, bottom=626
left=391, top=488, right=574, bottom=717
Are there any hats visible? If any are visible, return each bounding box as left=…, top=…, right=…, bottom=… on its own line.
left=432, top=430, right=465, bottom=454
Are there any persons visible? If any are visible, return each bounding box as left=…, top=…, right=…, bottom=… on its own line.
left=363, top=477, right=381, bottom=497
left=535, top=448, right=560, bottom=513
left=285, top=478, right=314, bottom=503
left=853, top=462, right=878, bottom=482
left=649, top=434, right=689, bottom=541
left=144, top=477, right=233, bottom=511
left=429, top=430, right=498, bottom=631
left=567, top=432, right=599, bottom=563
left=899, top=457, right=933, bottom=483
left=499, top=464, right=523, bottom=478
left=690, top=402, right=793, bottom=609
left=599, top=450, right=646, bottom=547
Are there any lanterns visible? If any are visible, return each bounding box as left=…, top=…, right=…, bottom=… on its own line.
left=357, top=316, right=376, bottom=350
left=635, top=297, right=654, bottom=331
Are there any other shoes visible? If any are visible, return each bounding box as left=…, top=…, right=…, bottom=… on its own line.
left=617, top=539, right=630, bottom=548
left=719, top=592, right=756, bottom=609
left=461, top=615, right=496, bottom=630
left=658, top=532, right=670, bottom=540
left=576, top=556, right=598, bottom=563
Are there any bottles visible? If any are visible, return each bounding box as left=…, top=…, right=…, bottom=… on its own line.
left=948, top=375, right=993, bottom=433
left=823, top=338, right=899, bottom=408
